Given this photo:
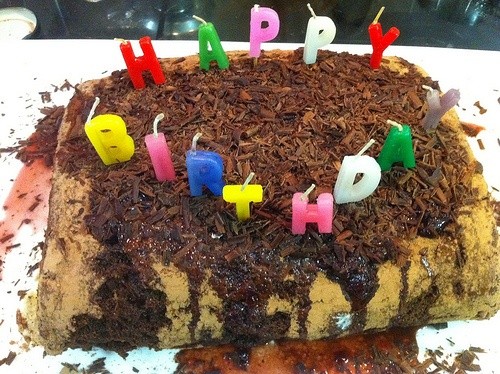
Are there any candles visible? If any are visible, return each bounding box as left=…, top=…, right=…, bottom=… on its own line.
left=420, top=84, right=461, bottom=132
left=192, top=15, right=230, bottom=70
left=185, top=132, right=226, bottom=198
left=114, top=37, right=165, bottom=90
left=333, top=138, right=381, bottom=204
left=367, top=7, right=401, bottom=69
left=144, top=114, right=178, bottom=182
left=250, top=4, right=280, bottom=66
left=302, top=3, right=336, bottom=63
left=83, top=96, right=134, bottom=166
left=377, top=119, right=417, bottom=171
left=291, top=184, right=333, bottom=234
left=222, top=171, right=264, bottom=220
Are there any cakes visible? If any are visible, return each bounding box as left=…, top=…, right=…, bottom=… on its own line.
left=15, top=47, right=500, bottom=355
